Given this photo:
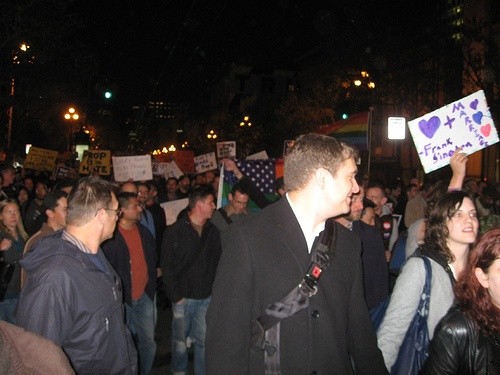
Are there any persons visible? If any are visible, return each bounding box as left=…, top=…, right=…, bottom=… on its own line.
left=328, top=147, right=500, bottom=375
left=13, top=174, right=139, bottom=375
left=203, top=132, right=393, bottom=375
left=0, top=154, right=293, bottom=291
left=99, top=191, right=160, bottom=375
left=160, top=186, right=223, bottom=375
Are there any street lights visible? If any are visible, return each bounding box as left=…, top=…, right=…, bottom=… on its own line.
left=240, top=115, right=252, bottom=153
left=0, top=38, right=36, bottom=155
left=207, top=129, right=217, bottom=151
left=64, top=106, right=80, bottom=152
left=354, top=70, right=383, bottom=147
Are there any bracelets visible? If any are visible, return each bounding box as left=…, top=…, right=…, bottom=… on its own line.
left=232, top=168, right=240, bottom=176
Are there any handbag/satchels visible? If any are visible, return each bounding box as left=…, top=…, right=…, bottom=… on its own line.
left=156, top=287, right=170, bottom=311
left=373, top=253, right=432, bottom=375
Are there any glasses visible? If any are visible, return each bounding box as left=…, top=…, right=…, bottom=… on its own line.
left=94, top=207, right=122, bottom=218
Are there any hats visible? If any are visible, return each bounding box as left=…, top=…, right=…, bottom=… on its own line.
left=118, top=191, right=138, bottom=204
